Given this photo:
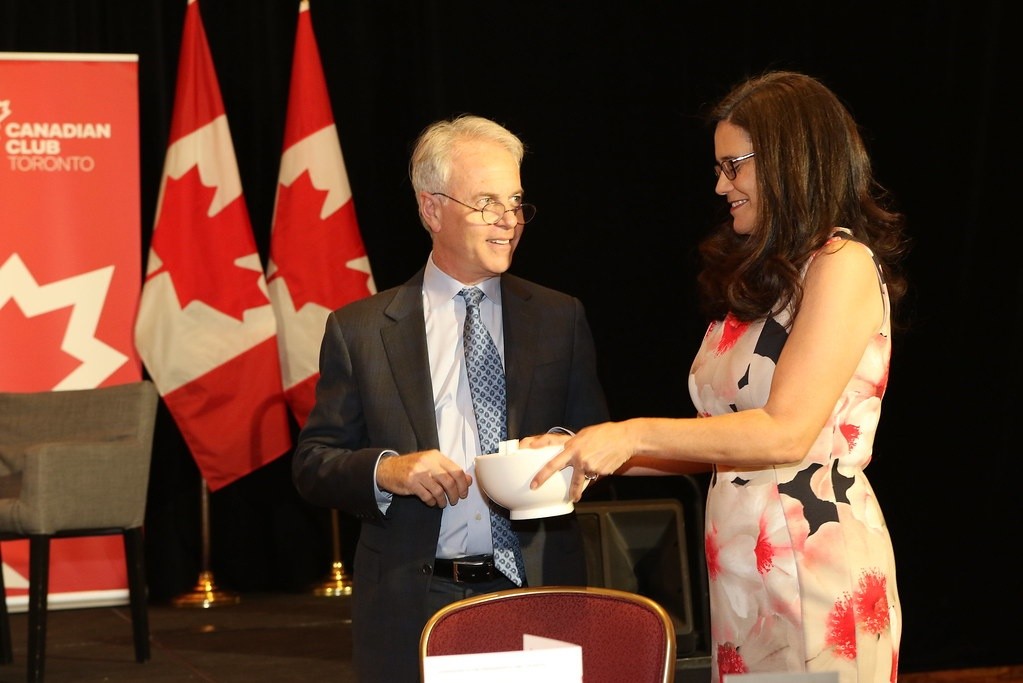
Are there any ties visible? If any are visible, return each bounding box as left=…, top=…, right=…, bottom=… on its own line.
left=456, top=284, right=527, bottom=588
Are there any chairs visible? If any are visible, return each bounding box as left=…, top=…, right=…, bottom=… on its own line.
left=420, top=584, right=676, bottom=683
left=0, top=379, right=159, bottom=683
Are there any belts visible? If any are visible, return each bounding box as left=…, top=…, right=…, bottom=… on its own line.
left=431, top=558, right=505, bottom=584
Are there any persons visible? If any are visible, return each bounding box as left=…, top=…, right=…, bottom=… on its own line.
left=517, top=72, right=903, bottom=683
left=292, top=117, right=611, bottom=683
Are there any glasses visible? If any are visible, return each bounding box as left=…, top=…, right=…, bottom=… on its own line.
left=432, top=192, right=538, bottom=225
left=712, top=152, right=756, bottom=181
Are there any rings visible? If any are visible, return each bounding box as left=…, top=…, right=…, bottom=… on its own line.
left=585, top=473, right=598, bottom=480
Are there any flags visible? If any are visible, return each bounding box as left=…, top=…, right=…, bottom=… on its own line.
left=265, top=0, right=378, bottom=428
left=133, top=0, right=292, bottom=493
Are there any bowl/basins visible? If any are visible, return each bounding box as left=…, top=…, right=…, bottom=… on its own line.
left=476, top=447, right=590, bottom=520
